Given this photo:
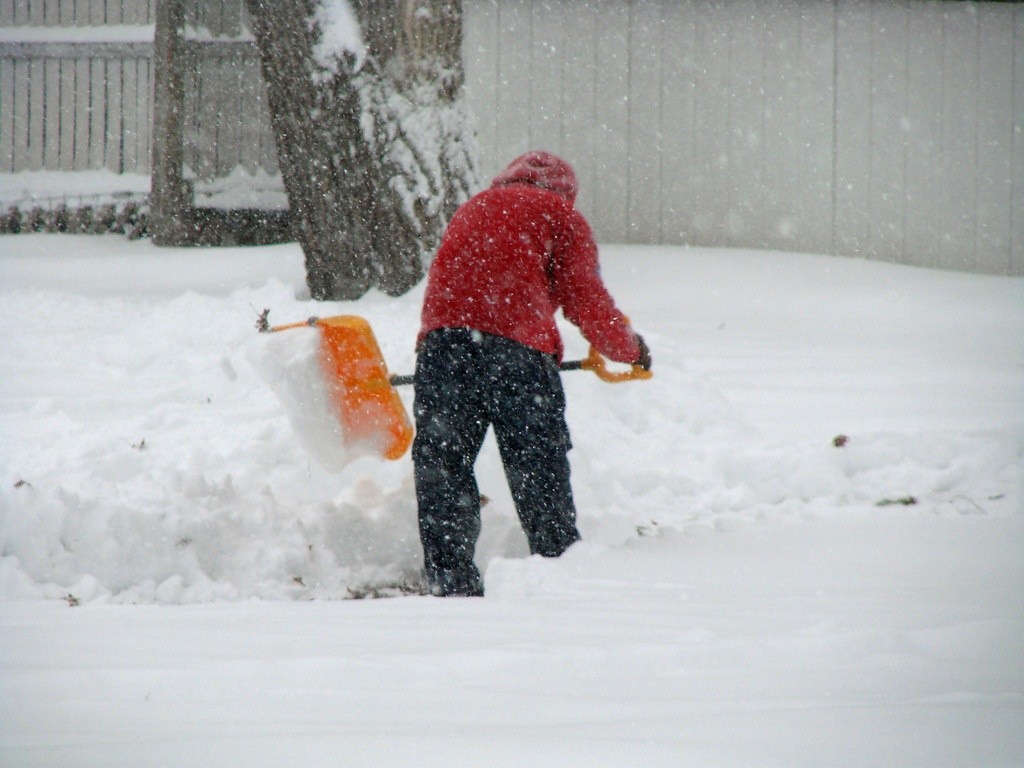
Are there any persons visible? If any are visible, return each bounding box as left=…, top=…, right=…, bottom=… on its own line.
left=413, top=149, right=652, bottom=597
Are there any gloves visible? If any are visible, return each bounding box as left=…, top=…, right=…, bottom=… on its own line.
left=633, top=334, right=651, bottom=371
